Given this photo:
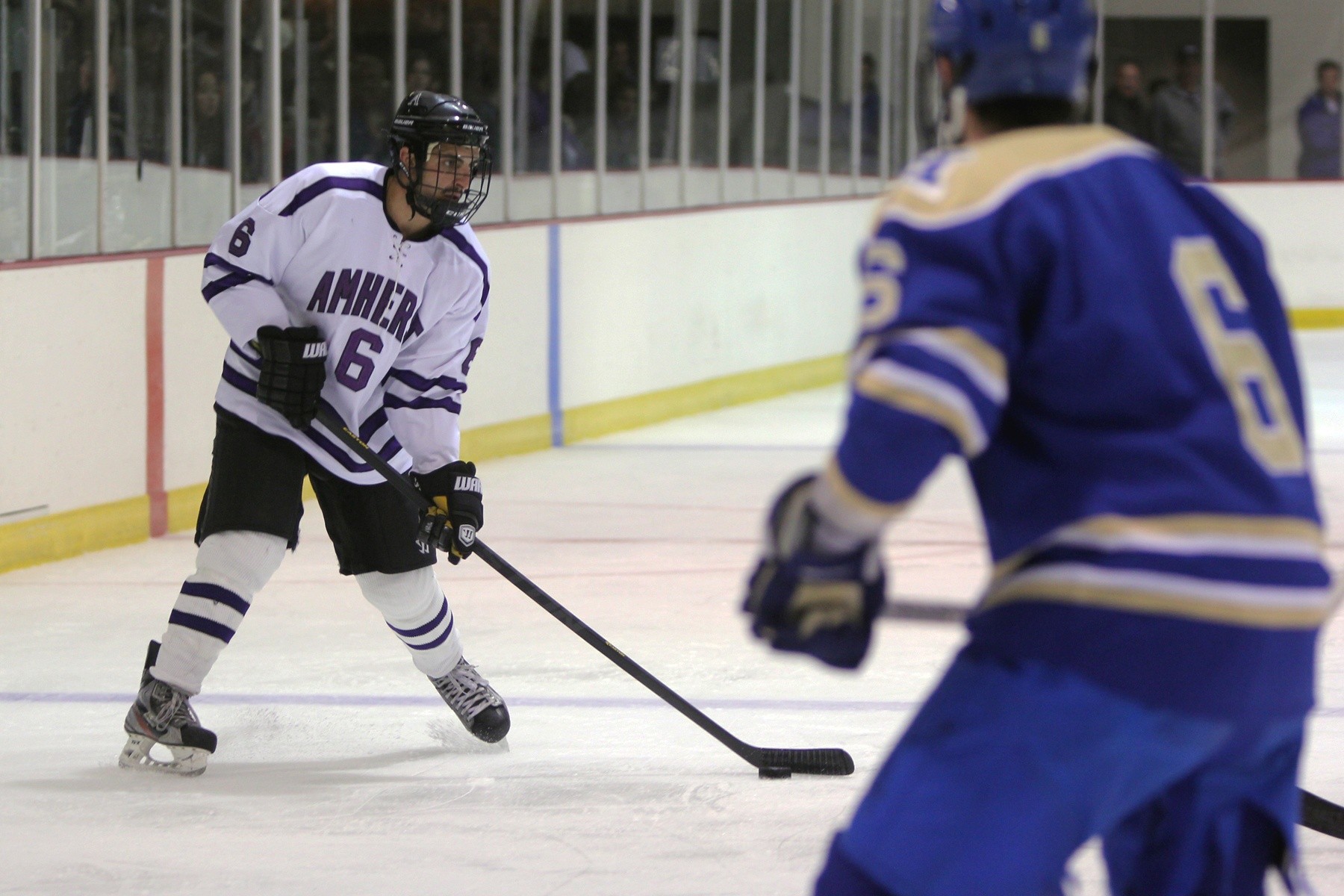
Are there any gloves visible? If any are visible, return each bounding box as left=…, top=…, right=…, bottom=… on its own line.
left=408, top=460, right=484, bottom=565
left=256, top=325, right=329, bottom=432
left=743, top=477, right=886, bottom=670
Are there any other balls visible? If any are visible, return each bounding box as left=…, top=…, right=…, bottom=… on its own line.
left=758, top=766, right=791, bottom=781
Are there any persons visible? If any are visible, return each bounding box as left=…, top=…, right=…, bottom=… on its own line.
left=1105, top=59, right=1197, bottom=175
left=1296, top=60, right=1344, bottom=179
left=407, top=51, right=434, bottom=91
left=192, top=67, right=223, bottom=168
left=1159, top=42, right=1238, bottom=177
left=118, top=91, right=511, bottom=777
left=860, top=55, right=879, bottom=131
left=739, top=1, right=1344, bottom=896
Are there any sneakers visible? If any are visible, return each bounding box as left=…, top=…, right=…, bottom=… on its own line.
left=428, top=656, right=511, bottom=752
left=119, top=640, right=217, bottom=777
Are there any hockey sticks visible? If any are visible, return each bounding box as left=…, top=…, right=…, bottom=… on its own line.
left=316, top=398, right=857, bottom=775
left=1294, top=786, right=1344, bottom=839
left=876, top=597, right=973, bottom=623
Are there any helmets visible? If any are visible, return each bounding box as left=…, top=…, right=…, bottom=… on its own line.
left=386, top=90, right=493, bottom=228
left=921, top=0, right=1098, bottom=143
